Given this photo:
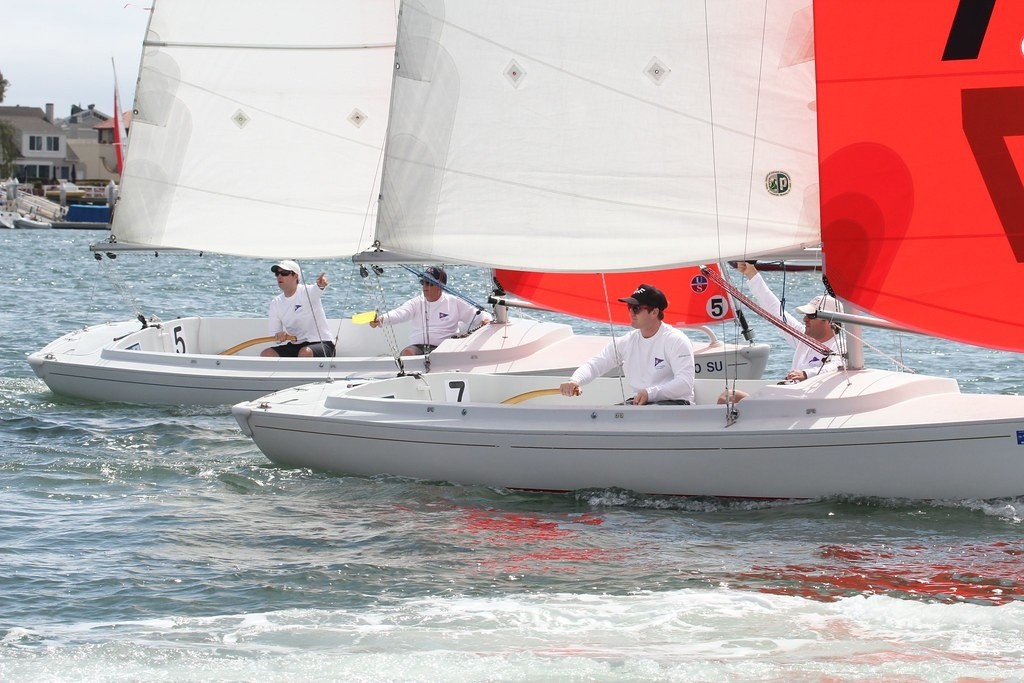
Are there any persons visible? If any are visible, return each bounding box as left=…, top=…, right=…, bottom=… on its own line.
left=559, top=284, right=696, bottom=406
left=370, top=266, right=493, bottom=356
left=717, top=262, right=849, bottom=404
left=0, top=195, right=36, bottom=220
left=260, top=260, right=336, bottom=357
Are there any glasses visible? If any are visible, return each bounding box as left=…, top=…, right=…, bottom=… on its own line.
left=806, top=314, right=817, bottom=319
left=420, top=280, right=434, bottom=286
left=627, top=303, right=649, bottom=314
left=274, top=270, right=295, bottom=276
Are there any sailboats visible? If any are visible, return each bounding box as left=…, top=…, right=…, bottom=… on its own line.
left=231, top=0, right=1024, bottom=503
left=22, top=2, right=773, bottom=405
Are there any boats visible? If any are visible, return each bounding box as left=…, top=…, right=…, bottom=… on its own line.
left=0, top=172, right=121, bottom=230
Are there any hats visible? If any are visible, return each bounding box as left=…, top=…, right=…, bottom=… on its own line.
left=270, top=260, right=302, bottom=283
left=796, top=295, right=844, bottom=322
left=618, top=284, right=668, bottom=310
left=418, top=266, right=447, bottom=284
